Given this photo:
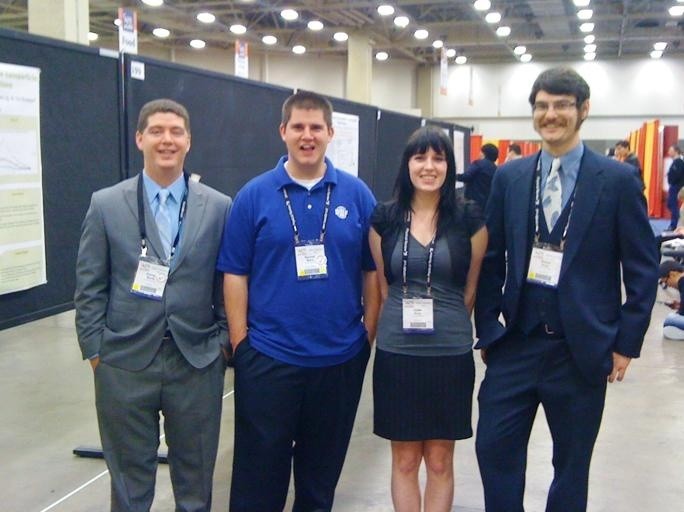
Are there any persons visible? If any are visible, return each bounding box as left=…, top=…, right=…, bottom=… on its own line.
left=505, top=145, right=521, bottom=160
left=368, top=126, right=489, bottom=512
left=216, top=92, right=382, bottom=512
left=75, top=97, right=232, bottom=512
left=474, top=65, right=662, bottom=512
left=455, top=143, right=499, bottom=201
left=660, top=261, right=684, bottom=342
left=615, top=141, right=646, bottom=192
left=665, top=145, right=684, bottom=231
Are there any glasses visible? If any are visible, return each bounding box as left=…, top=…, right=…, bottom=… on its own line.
left=533, top=100, right=575, bottom=112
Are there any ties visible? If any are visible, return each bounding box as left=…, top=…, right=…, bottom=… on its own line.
left=542, top=158, right=562, bottom=235
left=156, top=189, right=171, bottom=262
